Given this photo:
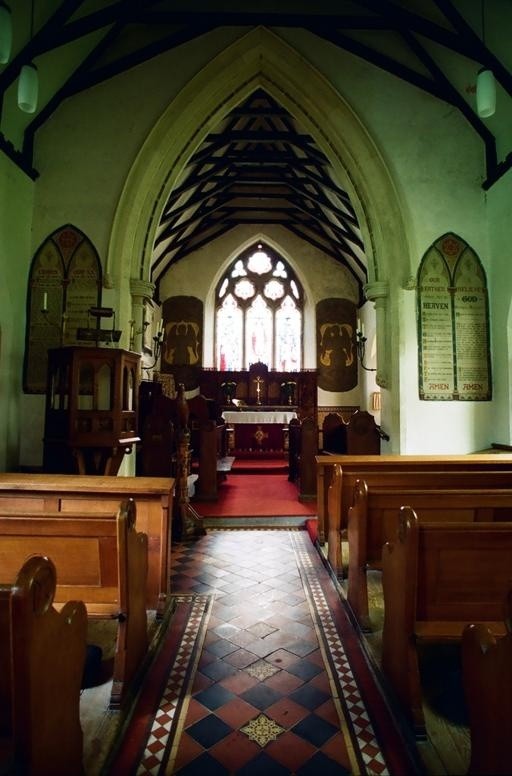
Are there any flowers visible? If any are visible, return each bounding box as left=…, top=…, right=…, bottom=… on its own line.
left=221, top=382, right=237, bottom=395
left=281, top=382, right=297, bottom=395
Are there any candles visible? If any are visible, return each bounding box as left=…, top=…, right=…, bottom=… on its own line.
left=153, top=317, right=165, bottom=343
left=145, top=305, right=150, bottom=323
left=355, top=317, right=365, bottom=341
left=43, top=291, right=48, bottom=310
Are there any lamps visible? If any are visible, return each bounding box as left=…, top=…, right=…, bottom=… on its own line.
left=0, top=0, right=13, bottom=65
left=19, top=0, right=38, bottom=113
left=476, top=0, right=497, bottom=119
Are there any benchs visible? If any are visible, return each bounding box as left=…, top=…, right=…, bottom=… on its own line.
left=0, top=498, right=151, bottom=709
left=0, top=552, right=88, bottom=776
left=328, top=464, right=512, bottom=776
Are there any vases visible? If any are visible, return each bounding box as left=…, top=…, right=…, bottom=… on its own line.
left=226, top=395, right=231, bottom=406
left=288, top=396, right=292, bottom=405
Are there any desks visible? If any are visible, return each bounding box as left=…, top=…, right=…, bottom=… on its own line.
left=0, top=468, right=176, bottom=617
left=315, top=454, right=512, bottom=546
